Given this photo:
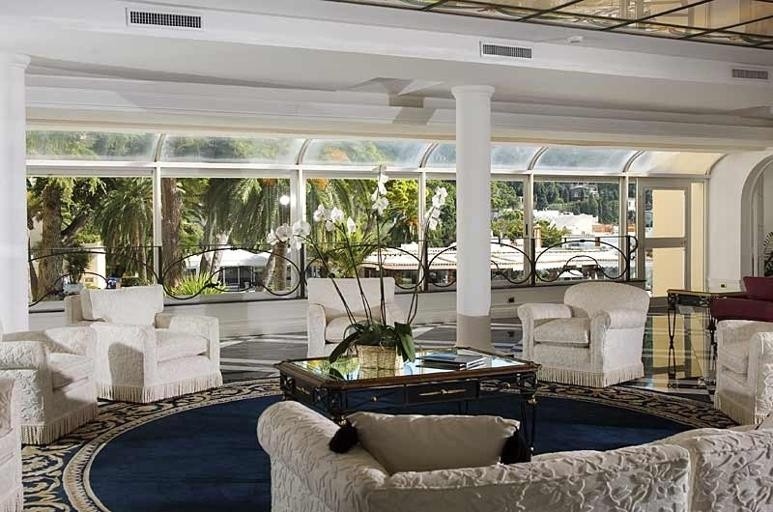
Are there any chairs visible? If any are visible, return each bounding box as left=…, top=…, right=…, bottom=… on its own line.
left=517, top=279, right=651, bottom=389
left=714, top=317, right=772, bottom=426
left=306, top=277, right=406, bottom=359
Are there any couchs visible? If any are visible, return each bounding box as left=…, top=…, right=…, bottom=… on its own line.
left=254, top=401, right=773, bottom=512
left=1, top=324, right=101, bottom=444
left=63, top=283, right=222, bottom=403
left=709, top=295, right=773, bottom=323
left=0, top=381, right=29, bottom=512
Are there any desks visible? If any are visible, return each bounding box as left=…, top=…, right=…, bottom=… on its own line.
left=666, top=288, right=746, bottom=389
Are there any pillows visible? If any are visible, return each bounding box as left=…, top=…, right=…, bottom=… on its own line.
left=743, top=275, right=773, bottom=299
left=344, top=409, right=520, bottom=474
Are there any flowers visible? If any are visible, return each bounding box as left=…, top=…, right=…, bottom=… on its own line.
left=263, top=164, right=448, bottom=360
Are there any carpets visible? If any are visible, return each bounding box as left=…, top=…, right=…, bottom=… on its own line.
left=21, top=378, right=741, bottom=511
